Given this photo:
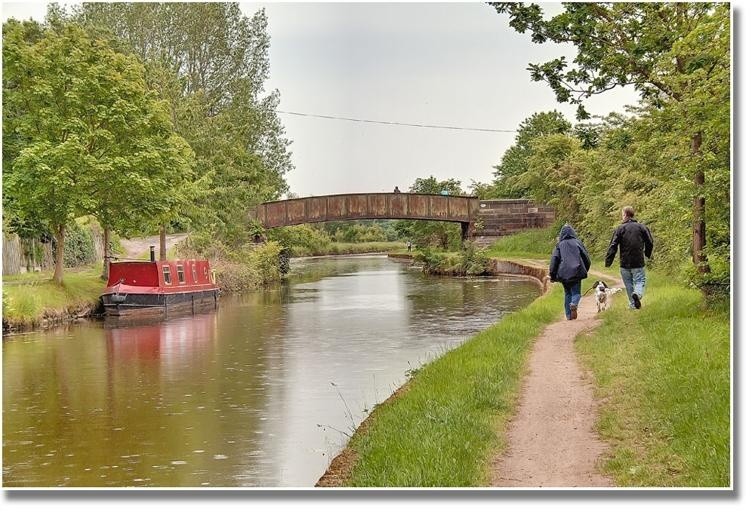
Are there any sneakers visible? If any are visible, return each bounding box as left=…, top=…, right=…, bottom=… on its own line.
left=633, top=294, right=641, bottom=309
left=570, top=303, right=577, bottom=319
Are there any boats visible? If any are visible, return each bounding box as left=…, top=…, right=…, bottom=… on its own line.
left=98, top=245, right=221, bottom=316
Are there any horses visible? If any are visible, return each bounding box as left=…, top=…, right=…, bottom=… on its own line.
left=593, top=281, right=609, bottom=313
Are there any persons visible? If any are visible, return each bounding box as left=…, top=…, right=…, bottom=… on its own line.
left=550, top=225, right=591, bottom=321
left=605, top=206, right=654, bottom=309
left=394, top=187, right=400, bottom=194
left=407, top=240, right=411, bottom=252
